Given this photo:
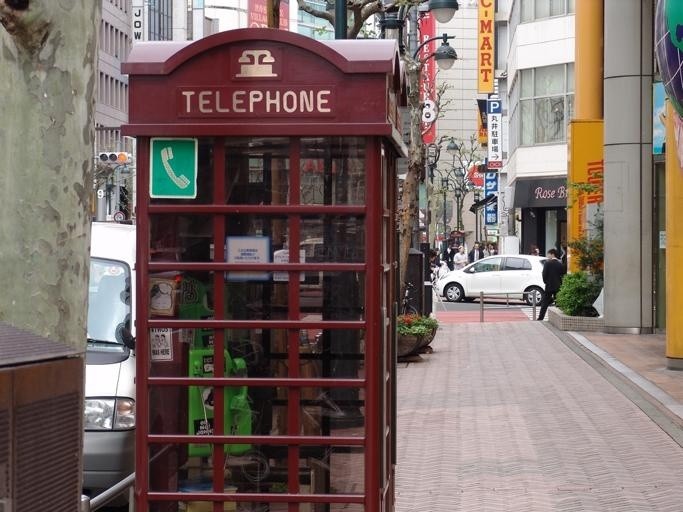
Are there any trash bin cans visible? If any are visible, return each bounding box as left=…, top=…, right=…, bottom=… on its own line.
left=408, top=249, right=426, bottom=316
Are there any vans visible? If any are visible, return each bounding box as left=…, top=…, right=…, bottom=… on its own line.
left=81, top=219, right=137, bottom=510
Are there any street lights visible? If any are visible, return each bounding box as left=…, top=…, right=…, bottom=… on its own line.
left=424, top=136, right=461, bottom=242
left=411, top=32, right=458, bottom=72
left=379, top=0, right=460, bottom=59
left=448, top=177, right=475, bottom=243
left=430, top=166, right=464, bottom=253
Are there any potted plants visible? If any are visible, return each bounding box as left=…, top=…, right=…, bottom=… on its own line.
left=401, top=312, right=437, bottom=355
left=398, top=318, right=422, bottom=363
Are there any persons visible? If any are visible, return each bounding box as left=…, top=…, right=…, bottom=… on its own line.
left=429, top=240, right=498, bottom=284
left=529, top=243, right=540, bottom=256
left=561, top=240, right=567, bottom=275
left=536, top=248, right=564, bottom=323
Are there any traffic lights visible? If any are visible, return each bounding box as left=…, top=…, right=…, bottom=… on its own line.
left=98, top=151, right=130, bottom=164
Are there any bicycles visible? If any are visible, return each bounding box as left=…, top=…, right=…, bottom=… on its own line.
left=399, top=281, right=420, bottom=317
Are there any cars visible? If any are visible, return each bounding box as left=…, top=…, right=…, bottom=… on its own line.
left=434, top=253, right=549, bottom=307
left=280, top=239, right=325, bottom=307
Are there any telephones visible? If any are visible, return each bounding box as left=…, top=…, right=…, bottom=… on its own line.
left=231, top=359, right=250, bottom=412
